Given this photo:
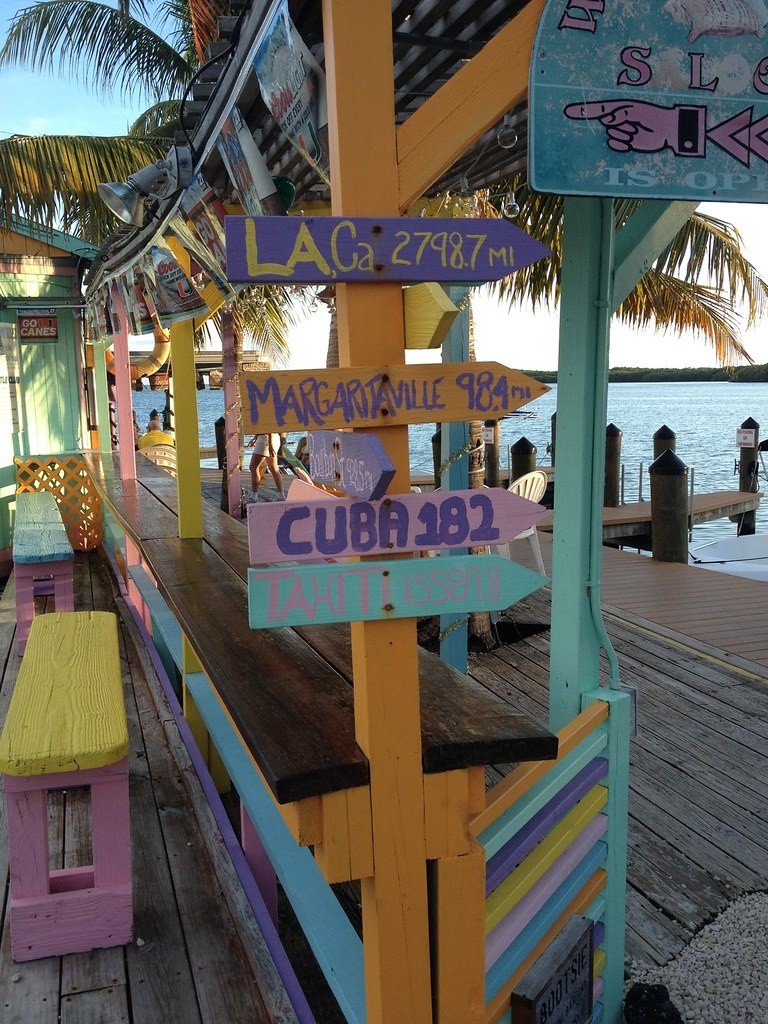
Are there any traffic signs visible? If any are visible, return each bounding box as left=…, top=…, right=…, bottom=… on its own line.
left=232, top=360, right=554, bottom=434
left=245, top=488, right=554, bottom=563
left=303, top=429, right=396, bottom=502
left=246, top=552, right=551, bottom=629
left=218, top=214, right=555, bottom=286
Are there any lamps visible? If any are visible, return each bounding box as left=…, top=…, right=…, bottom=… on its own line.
left=97, top=159, right=172, bottom=227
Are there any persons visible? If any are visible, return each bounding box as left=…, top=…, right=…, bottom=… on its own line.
left=138, top=420, right=175, bottom=450
left=244, top=433, right=288, bottom=508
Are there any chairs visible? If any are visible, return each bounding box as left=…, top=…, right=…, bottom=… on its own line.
left=487, top=469, right=549, bottom=579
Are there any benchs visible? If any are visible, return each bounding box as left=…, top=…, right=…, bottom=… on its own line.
left=0, top=610, right=136, bottom=963
left=12, top=491, right=75, bottom=657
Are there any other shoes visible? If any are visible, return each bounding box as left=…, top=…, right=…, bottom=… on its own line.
left=272, top=496, right=287, bottom=502
left=242, top=496, right=261, bottom=508
